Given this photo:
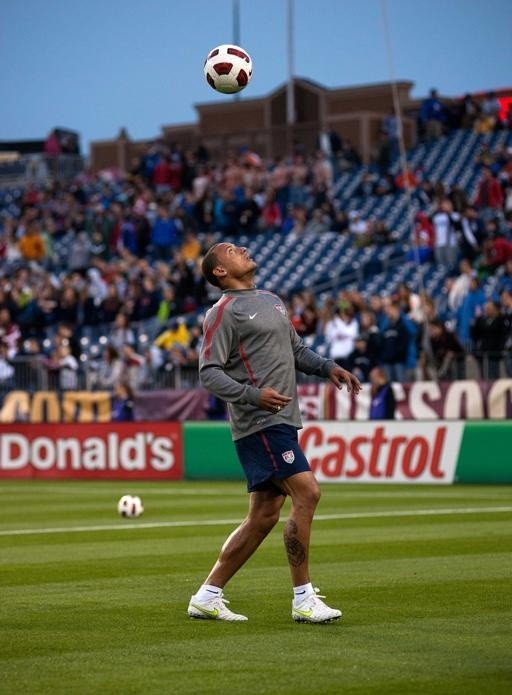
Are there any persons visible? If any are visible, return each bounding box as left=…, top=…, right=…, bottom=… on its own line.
left=108, top=383, right=134, bottom=421
left=368, top=366, right=397, bottom=419
left=185, top=240, right=363, bottom=625
left=202, top=391, right=229, bottom=420
left=1, top=88, right=511, bottom=389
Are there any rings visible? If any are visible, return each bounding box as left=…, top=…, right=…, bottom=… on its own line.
left=277, top=405, right=280, bottom=412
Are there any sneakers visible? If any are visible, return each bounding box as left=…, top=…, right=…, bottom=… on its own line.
left=187, top=592, right=249, bottom=621
left=291, top=586, right=342, bottom=623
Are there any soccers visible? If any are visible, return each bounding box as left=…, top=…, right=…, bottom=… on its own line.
left=116, top=494, right=143, bottom=517
left=203, top=44, right=252, bottom=93
